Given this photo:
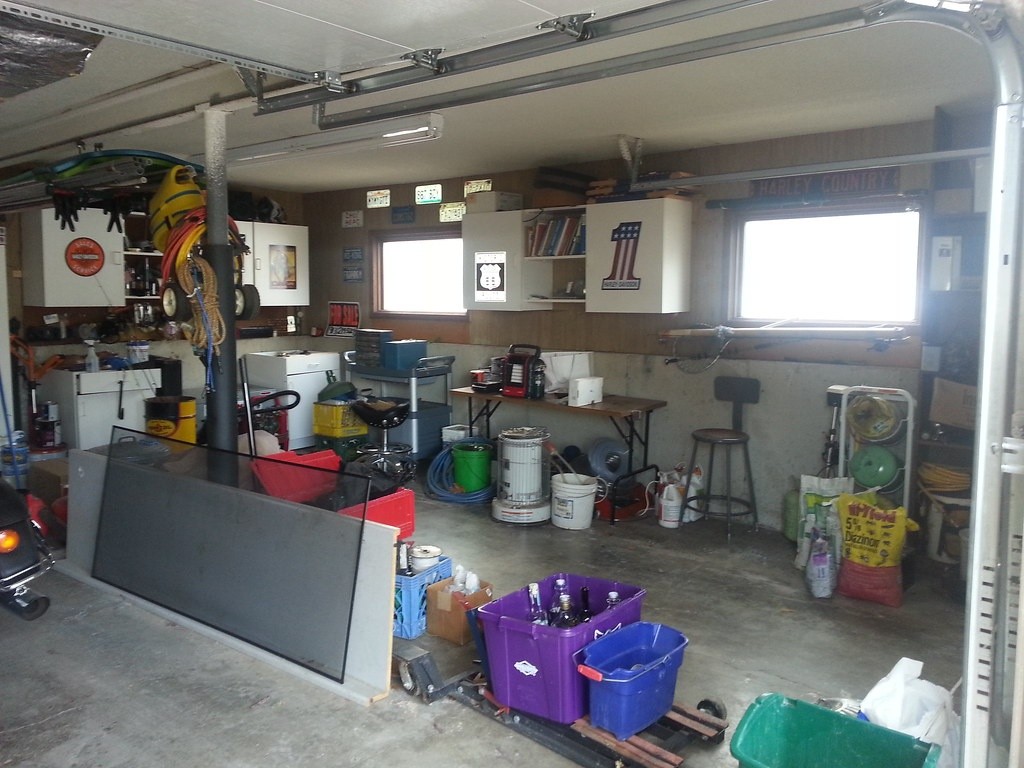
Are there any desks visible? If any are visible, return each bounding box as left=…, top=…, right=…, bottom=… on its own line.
left=448, top=385, right=667, bottom=525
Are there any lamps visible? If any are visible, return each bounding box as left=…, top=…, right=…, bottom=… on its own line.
left=185, top=111, right=444, bottom=171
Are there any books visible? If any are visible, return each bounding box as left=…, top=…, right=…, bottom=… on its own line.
left=528, top=211, right=587, bottom=257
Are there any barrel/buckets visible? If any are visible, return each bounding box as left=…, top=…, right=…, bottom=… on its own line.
left=2, top=401, right=69, bottom=498
left=682, top=472, right=705, bottom=522
left=142, top=394, right=197, bottom=451
left=125, top=340, right=149, bottom=364
left=451, top=442, right=494, bottom=493
left=409, top=545, right=442, bottom=576
left=550, top=472, right=599, bottom=531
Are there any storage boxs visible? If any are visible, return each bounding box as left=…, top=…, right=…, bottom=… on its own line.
left=30, top=457, right=68, bottom=497
left=927, top=376, right=977, bottom=432
left=393, top=556, right=648, bottom=724
left=567, top=376, right=604, bottom=407
left=465, top=192, right=523, bottom=212
left=384, top=339, right=429, bottom=370
left=250, top=451, right=416, bottom=538
left=313, top=401, right=480, bottom=462
left=532, top=165, right=597, bottom=206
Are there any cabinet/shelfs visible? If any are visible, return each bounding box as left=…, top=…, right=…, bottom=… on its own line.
left=461, top=197, right=693, bottom=314
left=344, top=350, right=454, bottom=465
left=20, top=205, right=310, bottom=307
left=41, top=368, right=162, bottom=450
left=245, top=350, right=340, bottom=452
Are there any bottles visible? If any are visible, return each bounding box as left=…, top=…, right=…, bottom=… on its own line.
left=527, top=578, right=620, bottom=630
left=657, top=481, right=682, bottom=528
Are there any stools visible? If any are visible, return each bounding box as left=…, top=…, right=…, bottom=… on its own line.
left=678, top=376, right=759, bottom=544
left=351, top=400, right=419, bottom=482
